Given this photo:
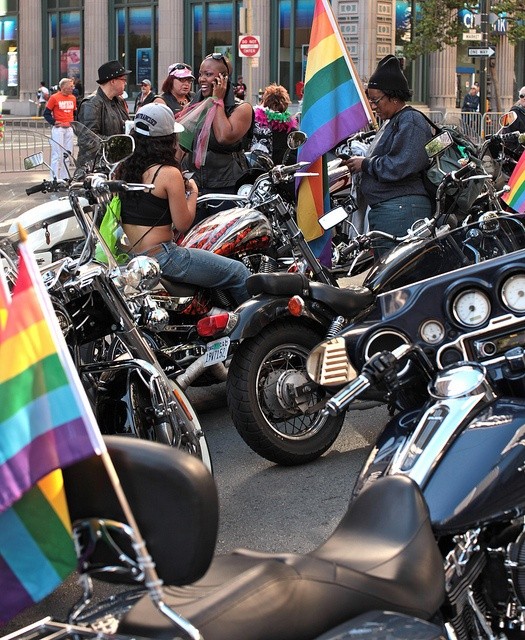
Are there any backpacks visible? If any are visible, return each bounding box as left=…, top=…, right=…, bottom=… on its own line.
left=95, top=163, right=168, bottom=266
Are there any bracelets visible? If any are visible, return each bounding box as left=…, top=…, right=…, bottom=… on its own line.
left=186, top=190, right=199, bottom=195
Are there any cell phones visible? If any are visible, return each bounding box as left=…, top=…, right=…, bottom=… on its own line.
left=183, top=171, right=196, bottom=181
left=338, top=154, right=350, bottom=161
left=212, top=72, right=225, bottom=87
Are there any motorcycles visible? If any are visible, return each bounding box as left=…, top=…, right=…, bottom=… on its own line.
left=326, top=128, right=376, bottom=279
left=196, top=130, right=525, bottom=465
left=0, top=343, right=524, bottom=640
left=0, top=122, right=213, bottom=478
left=14, top=131, right=339, bottom=391
left=461, top=110, right=524, bottom=224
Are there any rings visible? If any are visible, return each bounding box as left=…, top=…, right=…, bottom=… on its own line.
left=218, top=83, right=222, bottom=85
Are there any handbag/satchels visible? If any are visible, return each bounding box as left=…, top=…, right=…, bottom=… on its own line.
left=424, top=124, right=485, bottom=213
left=42, top=94, right=49, bottom=100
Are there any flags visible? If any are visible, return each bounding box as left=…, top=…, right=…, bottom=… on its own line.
left=294, top=0, right=373, bottom=271
left=501, top=150, right=525, bottom=213
left=1, top=241, right=106, bottom=629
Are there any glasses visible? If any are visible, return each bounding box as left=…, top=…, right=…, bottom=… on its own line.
left=520, top=95, right=524, bottom=98
left=205, top=52, right=230, bottom=74
left=174, top=77, right=193, bottom=84
left=372, top=94, right=384, bottom=105
left=118, top=78, right=126, bottom=81
left=141, top=84, right=147, bottom=87
left=169, top=64, right=192, bottom=75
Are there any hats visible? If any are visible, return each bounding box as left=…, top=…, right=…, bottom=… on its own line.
left=168, top=63, right=195, bottom=79
left=96, top=61, right=132, bottom=84
left=141, top=79, right=151, bottom=86
left=367, top=55, right=410, bottom=90
left=134, top=103, right=185, bottom=137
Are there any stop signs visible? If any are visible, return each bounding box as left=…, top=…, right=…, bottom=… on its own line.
left=239, top=35, right=260, bottom=57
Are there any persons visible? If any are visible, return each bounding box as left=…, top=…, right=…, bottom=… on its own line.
left=36, top=81, right=49, bottom=116
left=345, top=54, right=433, bottom=263
left=72, top=77, right=82, bottom=108
left=73, top=60, right=134, bottom=180
left=237, top=82, right=299, bottom=174
left=234, top=75, right=246, bottom=100
left=133, top=79, right=156, bottom=121
left=174, top=51, right=255, bottom=235
left=111, top=102, right=252, bottom=306
left=44, top=78, right=77, bottom=180
left=459, top=80, right=481, bottom=137
left=153, top=62, right=196, bottom=116
left=501, top=86, right=525, bottom=177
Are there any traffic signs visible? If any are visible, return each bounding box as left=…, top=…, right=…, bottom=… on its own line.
left=468, top=46, right=495, bottom=58
left=463, top=33, right=483, bottom=40
